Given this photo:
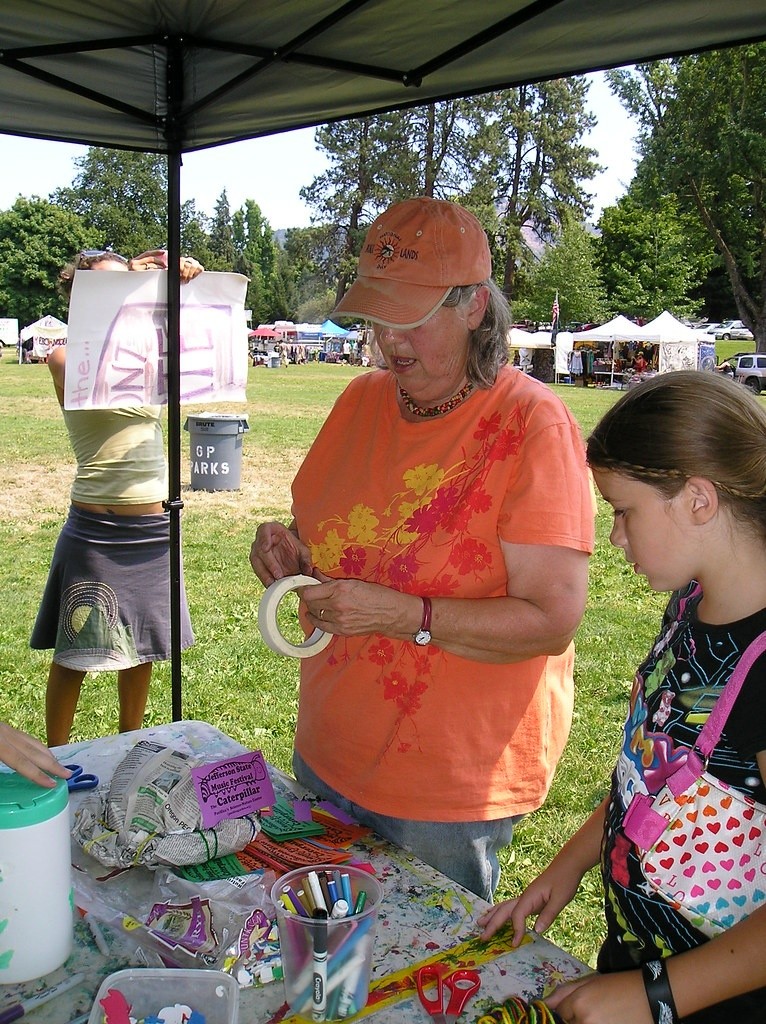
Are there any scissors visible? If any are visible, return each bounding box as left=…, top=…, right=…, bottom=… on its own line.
left=416, top=967, right=482, bottom=1024
left=62, top=764, right=99, bottom=792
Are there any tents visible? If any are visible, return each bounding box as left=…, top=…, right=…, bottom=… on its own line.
left=640, top=310, right=698, bottom=376
left=572, top=315, right=661, bottom=389
left=318, top=319, right=350, bottom=335
left=506, top=328, right=553, bottom=349
left=19, top=314, right=68, bottom=364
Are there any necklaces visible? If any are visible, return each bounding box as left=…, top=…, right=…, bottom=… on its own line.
left=398, top=380, right=474, bottom=416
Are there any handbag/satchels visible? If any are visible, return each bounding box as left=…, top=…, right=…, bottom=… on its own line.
left=626, top=630, right=766, bottom=938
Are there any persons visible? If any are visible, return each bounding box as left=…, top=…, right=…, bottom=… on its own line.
left=0, top=724, right=73, bottom=789
left=274, top=340, right=289, bottom=368
left=714, top=359, right=732, bottom=378
left=342, top=339, right=372, bottom=365
left=631, top=351, right=644, bottom=375
left=31, top=250, right=204, bottom=748
left=513, top=350, right=520, bottom=367
left=477, top=373, right=766, bottom=1024
left=249, top=196, right=596, bottom=905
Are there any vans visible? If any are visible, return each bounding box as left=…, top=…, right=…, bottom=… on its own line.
left=726, top=353, right=766, bottom=396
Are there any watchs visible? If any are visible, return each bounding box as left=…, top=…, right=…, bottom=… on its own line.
left=412, top=596, right=433, bottom=646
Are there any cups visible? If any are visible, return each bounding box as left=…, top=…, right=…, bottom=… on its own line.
left=271, top=865, right=383, bottom=1021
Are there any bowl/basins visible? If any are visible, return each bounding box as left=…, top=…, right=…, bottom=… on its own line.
left=89, top=968, right=240, bottom=1024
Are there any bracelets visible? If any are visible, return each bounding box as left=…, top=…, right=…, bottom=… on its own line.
left=642, top=958, right=680, bottom=1024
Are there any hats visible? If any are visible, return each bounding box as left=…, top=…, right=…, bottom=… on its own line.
left=329, top=196, right=492, bottom=330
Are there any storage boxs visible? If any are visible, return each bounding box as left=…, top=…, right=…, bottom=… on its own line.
left=563, top=377, right=575, bottom=384
left=87, top=968, right=240, bottom=1024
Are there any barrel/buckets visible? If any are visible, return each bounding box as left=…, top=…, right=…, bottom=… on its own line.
left=0, top=771, right=73, bottom=985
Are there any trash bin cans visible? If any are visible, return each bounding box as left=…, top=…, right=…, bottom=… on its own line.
left=307, top=351, right=315, bottom=361
left=271, top=357, right=280, bottom=368
left=319, top=352, right=326, bottom=361
left=187, top=413, right=250, bottom=492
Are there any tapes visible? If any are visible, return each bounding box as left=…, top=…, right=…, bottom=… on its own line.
left=257, top=574, right=332, bottom=658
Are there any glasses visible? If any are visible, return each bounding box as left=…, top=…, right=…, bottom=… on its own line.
left=77, top=251, right=127, bottom=269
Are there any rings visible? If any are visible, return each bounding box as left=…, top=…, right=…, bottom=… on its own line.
left=186, top=260, right=192, bottom=264
left=145, top=263, right=148, bottom=269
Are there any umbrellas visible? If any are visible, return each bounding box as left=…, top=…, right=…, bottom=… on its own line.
left=249, top=328, right=282, bottom=336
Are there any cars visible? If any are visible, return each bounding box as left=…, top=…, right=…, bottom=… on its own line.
left=685, top=321, right=754, bottom=341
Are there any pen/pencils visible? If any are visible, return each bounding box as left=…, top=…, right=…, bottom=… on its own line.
left=0, top=973, right=87, bottom=1024
left=312, top=907, right=328, bottom=1022
left=77, top=907, right=109, bottom=956
left=278, top=869, right=373, bottom=1020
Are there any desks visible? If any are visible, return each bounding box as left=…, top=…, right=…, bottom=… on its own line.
left=593, top=372, right=624, bottom=382
left=0, top=718, right=591, bottom=1024
